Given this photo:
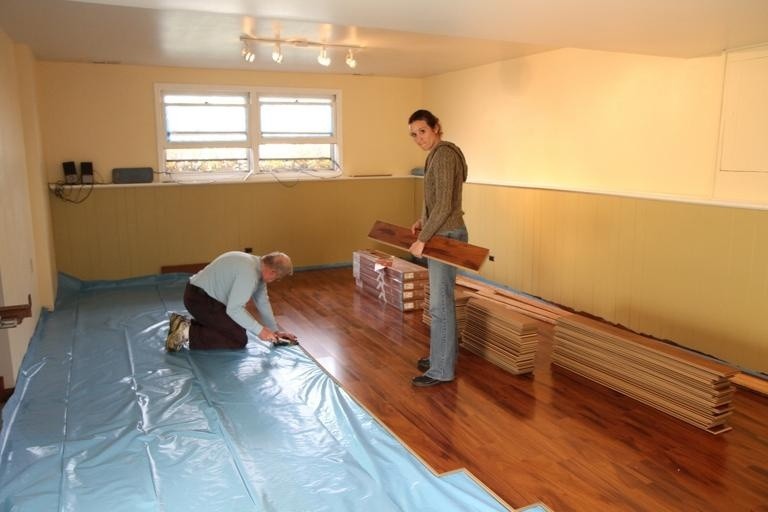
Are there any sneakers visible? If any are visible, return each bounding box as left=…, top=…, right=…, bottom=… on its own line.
left=166, top=313, right=188, bottom=353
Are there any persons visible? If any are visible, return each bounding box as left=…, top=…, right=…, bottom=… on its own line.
left=405, top=107, right=471, bottom=388
left=164, top=250, right=298, bottom=353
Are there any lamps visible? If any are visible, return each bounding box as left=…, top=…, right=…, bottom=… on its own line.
left=239, top=16, right=364, bottom=69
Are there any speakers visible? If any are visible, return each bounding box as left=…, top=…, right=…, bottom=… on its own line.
left=111, top=167, right=153, bottom=184
left=62, top=161, right=78, bottom=184
left=81, top=161, right=94, bottom=184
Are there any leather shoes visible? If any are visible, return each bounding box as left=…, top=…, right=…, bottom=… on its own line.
left=418, top=359, right=430, bottom=368
left=411, top=376, right=440, bottom=387
